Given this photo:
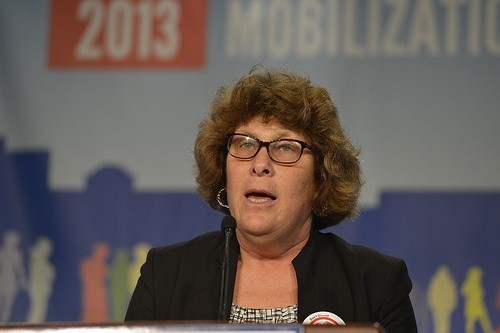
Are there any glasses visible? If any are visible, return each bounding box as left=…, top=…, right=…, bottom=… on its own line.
left=226, top=133, right=315, bottom=164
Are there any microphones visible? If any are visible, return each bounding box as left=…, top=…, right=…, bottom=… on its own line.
left=218, top=214, right=236, bottom=321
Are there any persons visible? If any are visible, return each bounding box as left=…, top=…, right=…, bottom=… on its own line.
left=125, top=60, right=420, bottom=333
